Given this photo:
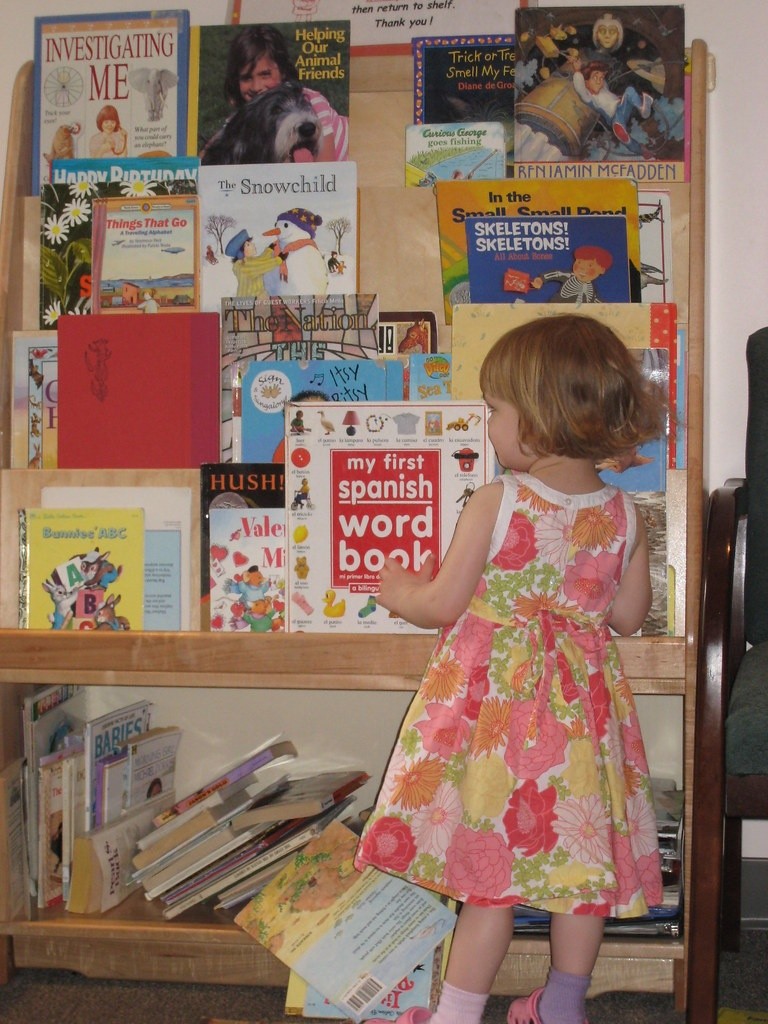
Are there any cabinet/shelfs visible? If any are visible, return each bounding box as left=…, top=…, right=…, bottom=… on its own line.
left=1, top=37, right=712, bottom=1010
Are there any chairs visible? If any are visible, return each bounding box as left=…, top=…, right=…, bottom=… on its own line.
left=685, top=325, right=768, bottom=1024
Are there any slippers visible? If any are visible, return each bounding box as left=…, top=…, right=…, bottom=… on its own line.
left=507, top=987, right=589, bottom=1024
left=363, top=1005, right=431, bottom=1024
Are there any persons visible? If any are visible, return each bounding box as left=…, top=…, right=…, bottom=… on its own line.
left=373, top=316, right=673, bottom=1024
left=228, top=25, right=348, bottom=160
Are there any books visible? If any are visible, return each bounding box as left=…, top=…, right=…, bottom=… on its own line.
left=20, top=684, right=683, bottom=1019
left=13, top=2, right=693, bottom=637
